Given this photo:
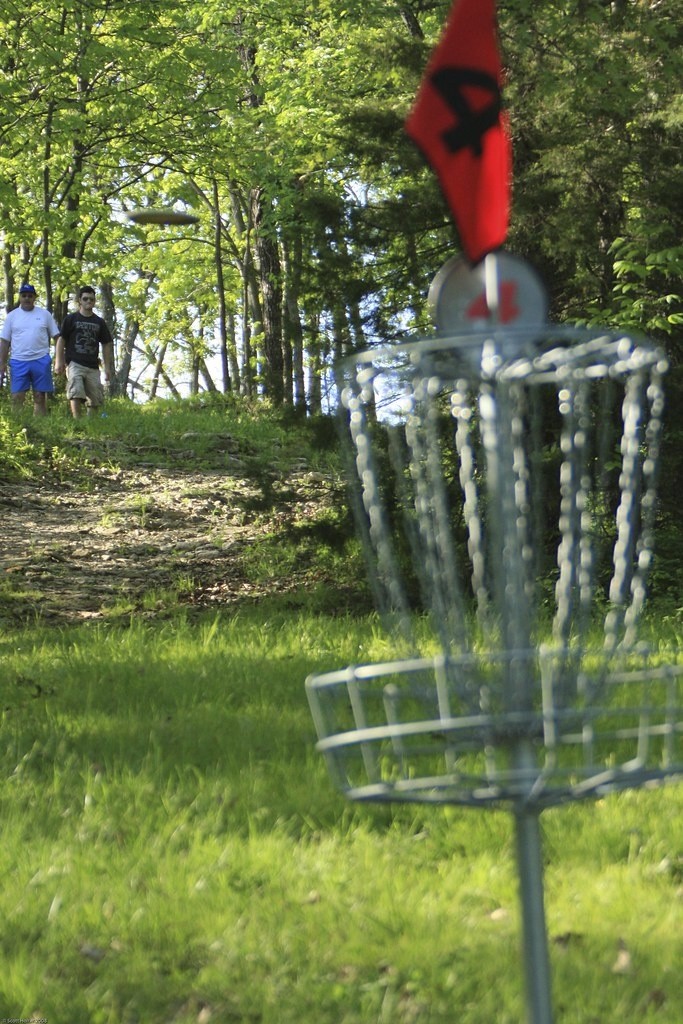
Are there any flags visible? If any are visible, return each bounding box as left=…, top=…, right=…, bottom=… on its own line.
left=406, top=0, right=513, bottom=272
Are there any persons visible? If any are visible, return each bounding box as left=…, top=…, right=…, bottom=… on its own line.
left=0, top=284, right=61, bottom=417
left=55, top=286, right=114, bottom=418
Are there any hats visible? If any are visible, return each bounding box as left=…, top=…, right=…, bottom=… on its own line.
left=20, top=284, right=35, bottom=293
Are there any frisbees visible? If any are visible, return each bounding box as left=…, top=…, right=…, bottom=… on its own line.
left=127, top=211, right=201, bottom=225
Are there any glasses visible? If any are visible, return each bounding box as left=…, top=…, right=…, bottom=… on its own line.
left=83, top=297, right=94, bottom=302
left=22, top=295, right=33, bottom=298
